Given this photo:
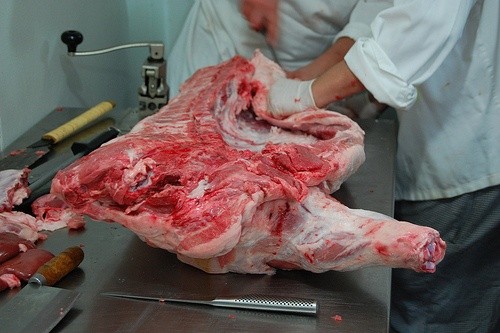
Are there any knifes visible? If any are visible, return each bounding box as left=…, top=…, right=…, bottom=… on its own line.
left=0, top=98, right=115, bottom=171
left=0, top=246, right=84, bottom=333
left=257, top=26, right=284, bottom=67
left=101, top=288, right=320, bottom=317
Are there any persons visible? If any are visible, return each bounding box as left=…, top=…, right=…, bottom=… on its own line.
left=266, top=0, right=500, bottom=333
left=165, top=0, right=390, bottom=119
